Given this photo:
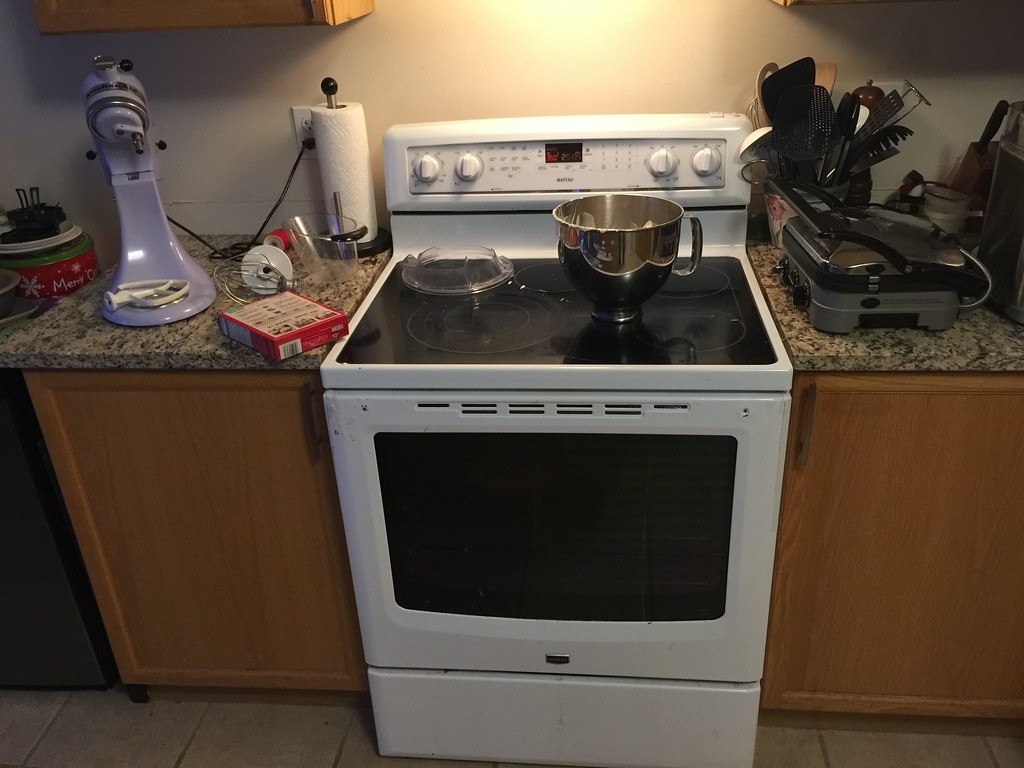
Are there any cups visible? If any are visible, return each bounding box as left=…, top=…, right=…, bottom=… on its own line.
left=281, top=213, right=369, bottom=283
left=552, top=194, right=703, bottom=323
left=762, top=181, right=848, bottom=249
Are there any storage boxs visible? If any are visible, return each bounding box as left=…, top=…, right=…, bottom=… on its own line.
left=217, top=289, right=350, bottom=363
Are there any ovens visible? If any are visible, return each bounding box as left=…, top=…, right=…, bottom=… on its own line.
left=322, top=392, right=792, bottom=768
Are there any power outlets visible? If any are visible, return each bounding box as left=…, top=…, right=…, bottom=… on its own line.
left=292, top=106, right=318, bottom=159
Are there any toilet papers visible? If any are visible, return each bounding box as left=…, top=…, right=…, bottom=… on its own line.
left=310, top=100, right=378, bottom=246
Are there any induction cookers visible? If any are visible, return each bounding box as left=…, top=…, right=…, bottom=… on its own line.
left=321, top=112, right=795, bottom=392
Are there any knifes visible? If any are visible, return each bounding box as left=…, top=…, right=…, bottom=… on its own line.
left=978, top=99, right=1009, bottom=156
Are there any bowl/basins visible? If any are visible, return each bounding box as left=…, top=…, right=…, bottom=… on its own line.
left=922, top=205, right=969, bottom=234
left=924, top=185, right=972, bottom=212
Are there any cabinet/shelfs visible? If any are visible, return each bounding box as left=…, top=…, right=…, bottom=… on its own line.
left=22, top=367, right=369, bottom=705
left=34, top=0, right=376, bottom=37
left=763, top=372, right=1024, bottom=721
